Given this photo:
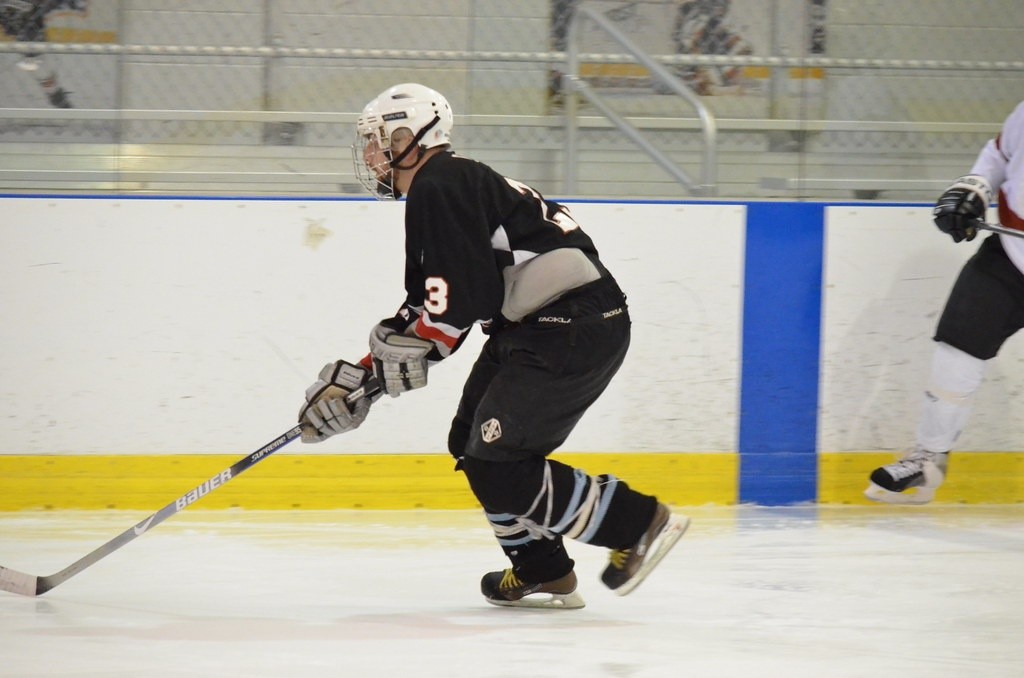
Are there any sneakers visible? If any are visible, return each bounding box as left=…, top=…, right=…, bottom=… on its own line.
left=602, top=501, right=690, bottom=596
left=481, top=558, right=585, bottom=608
left=864, top=447, right=949, bottom=504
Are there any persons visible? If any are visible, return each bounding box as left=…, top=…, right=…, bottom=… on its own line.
left=864, top=100, right=1024, bottom=505
left=0, top=0, right=90, bottom=109
left=545, top=0, right=758, bottom=112
left=298, top=83, right=689, bottom=611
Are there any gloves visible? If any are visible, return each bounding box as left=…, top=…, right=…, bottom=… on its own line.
left=298, top=359, right=384, bottom=442
left=368, top=324, right=435, bottom=398
left=933, top=176, right=993, bottom=243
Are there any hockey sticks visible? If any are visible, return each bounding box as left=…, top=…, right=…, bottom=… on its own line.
left=0, top=376, right=383, bottom=599
left=966, top=216, right=1024, bottom=239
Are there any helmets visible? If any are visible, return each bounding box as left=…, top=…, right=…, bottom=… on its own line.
left=350, top=83, right=453, bottom=201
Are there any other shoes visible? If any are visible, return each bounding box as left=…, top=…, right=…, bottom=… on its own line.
left=50, top=88, right=73, bottom=107
